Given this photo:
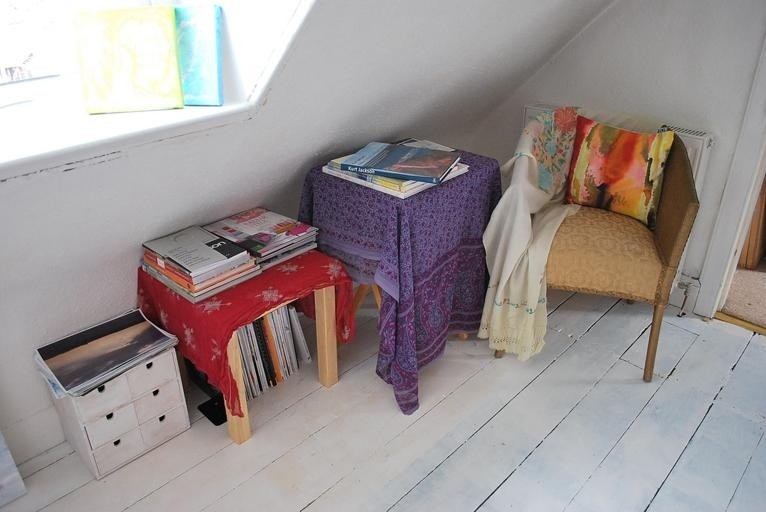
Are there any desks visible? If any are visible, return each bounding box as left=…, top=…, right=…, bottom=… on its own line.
left=139, top=251, right=345, bottom=447
left=312, top=138, right=503, bottom=340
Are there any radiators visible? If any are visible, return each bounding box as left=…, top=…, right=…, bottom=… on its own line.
left=522, top=103, right=715, bottom=295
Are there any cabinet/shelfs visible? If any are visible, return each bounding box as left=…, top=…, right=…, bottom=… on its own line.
left=46, top=350, right=193, bottom=482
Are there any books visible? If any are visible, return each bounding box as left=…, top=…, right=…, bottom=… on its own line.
left=140, top=205, right=320, bottom=305
left=320, top=134, right=472, bottom=201
left=181, top=304, right=313, bottom=406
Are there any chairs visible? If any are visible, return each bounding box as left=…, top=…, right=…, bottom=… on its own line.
left=493, top=107, right=701, bottom=385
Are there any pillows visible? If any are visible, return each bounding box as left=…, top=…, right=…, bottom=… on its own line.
left=561, top=115, right=675, bottom=231
